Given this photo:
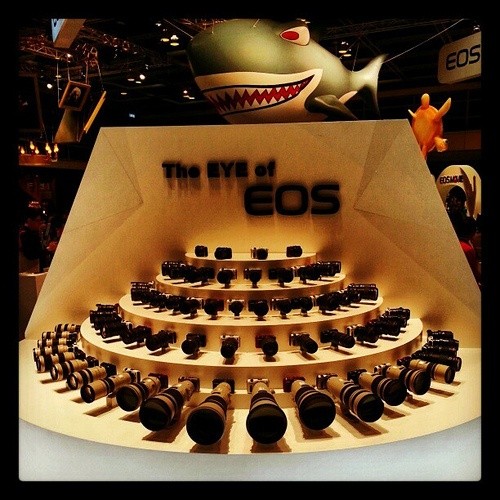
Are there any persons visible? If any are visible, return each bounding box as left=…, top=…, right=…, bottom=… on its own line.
left=18, top=209, right=56, bottom=273
left=445, top=185, right=481, bottom=292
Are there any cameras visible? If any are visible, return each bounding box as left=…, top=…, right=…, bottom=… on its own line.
left=32, top=244, right=462, bottom=446
left=446, top=195, right=456, bottom=206
